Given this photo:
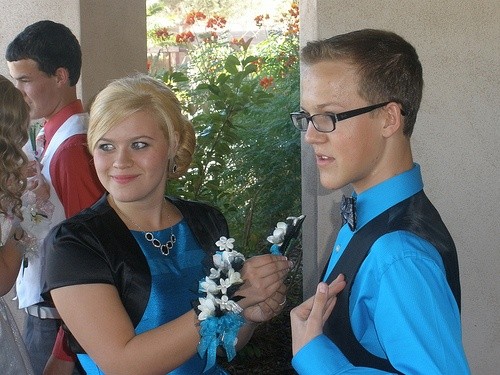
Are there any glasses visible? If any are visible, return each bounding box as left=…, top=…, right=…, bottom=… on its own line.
left=290, top=100, right=409, bottom=133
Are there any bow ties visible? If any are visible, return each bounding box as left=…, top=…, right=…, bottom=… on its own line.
left=340, top=194, right=357, bottom=232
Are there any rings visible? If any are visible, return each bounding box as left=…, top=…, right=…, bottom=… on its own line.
left=279, top=296, right=287, bottom=306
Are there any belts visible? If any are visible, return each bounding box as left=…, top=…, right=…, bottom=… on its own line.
left=24, top=305, right=63, bottom=320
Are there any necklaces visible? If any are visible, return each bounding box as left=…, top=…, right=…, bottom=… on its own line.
left=115, top=197, right=176, bottom=256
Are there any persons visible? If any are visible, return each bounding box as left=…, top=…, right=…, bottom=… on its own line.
left=0, top=74, right=52, bottom=298
left=289, top=28, right=472, bottom=375
left=4, top=18, right=107, bottom=375
left=38, top=70, right=296, bottom=375
